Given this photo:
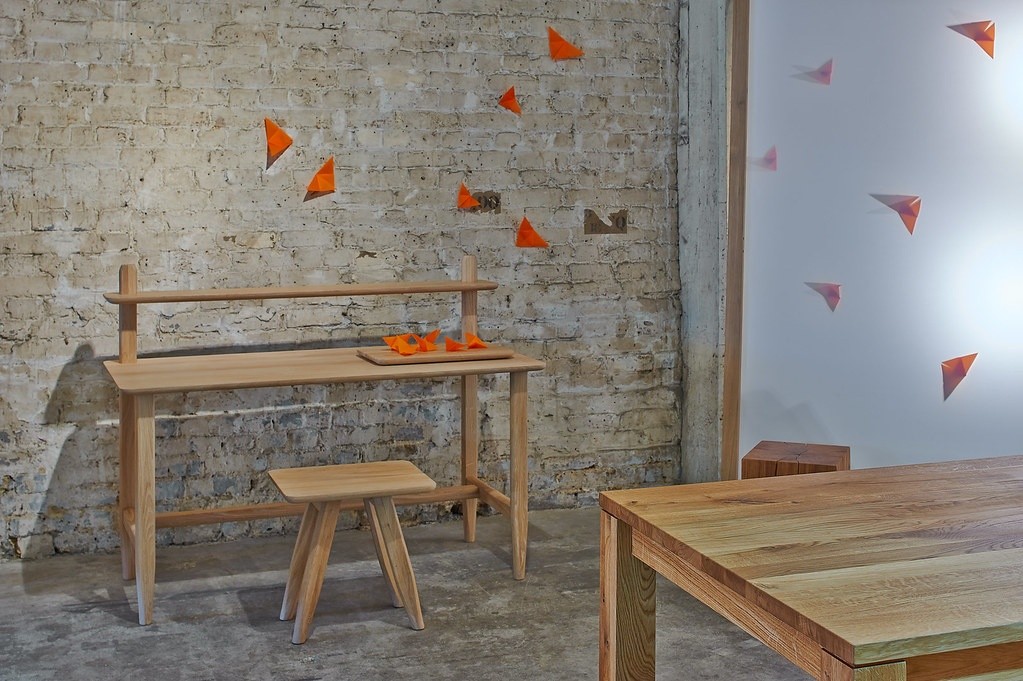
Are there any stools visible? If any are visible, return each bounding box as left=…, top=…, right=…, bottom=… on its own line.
left=742, top=441, right=852, bottom=481
left=268, top=458, right=437, bottom=645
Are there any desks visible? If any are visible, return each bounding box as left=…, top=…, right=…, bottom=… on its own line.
left=598, top=452, right=1023, bottom=681
left=103, top=257, right=546, bottom=625
